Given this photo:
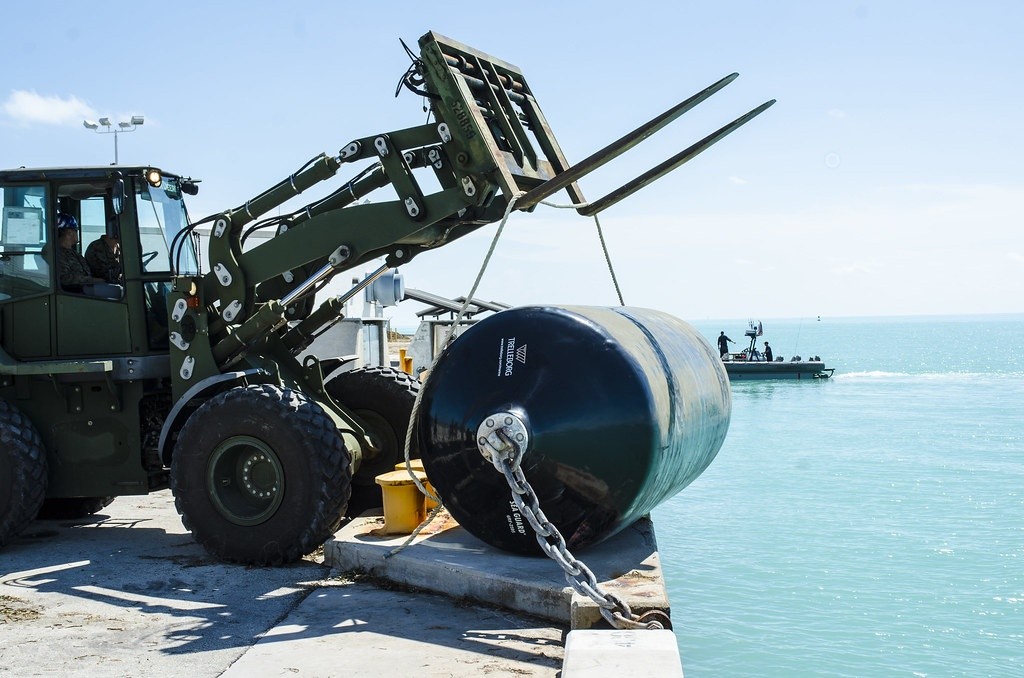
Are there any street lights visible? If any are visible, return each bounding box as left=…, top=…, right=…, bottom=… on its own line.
left=80, top=113, right=144, bottom=167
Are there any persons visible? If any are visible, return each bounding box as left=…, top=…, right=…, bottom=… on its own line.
left=54, top=212, right=121, bottom=298
left=763, top=342, right=773, bottom=361
left=718, top=332, right=736, bottom=361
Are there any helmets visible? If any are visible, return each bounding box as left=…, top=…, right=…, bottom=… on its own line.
left=56, top=212, right=80, bottom=231
left=764, top=342, right=769, bottom=344
left=106, top=215, right=120, bottom=240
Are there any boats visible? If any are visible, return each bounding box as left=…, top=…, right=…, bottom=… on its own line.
left=718, top=320, right=836, bottom=378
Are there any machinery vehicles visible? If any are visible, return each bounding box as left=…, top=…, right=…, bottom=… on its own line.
left=0, top=28, right=779, bottom=565
